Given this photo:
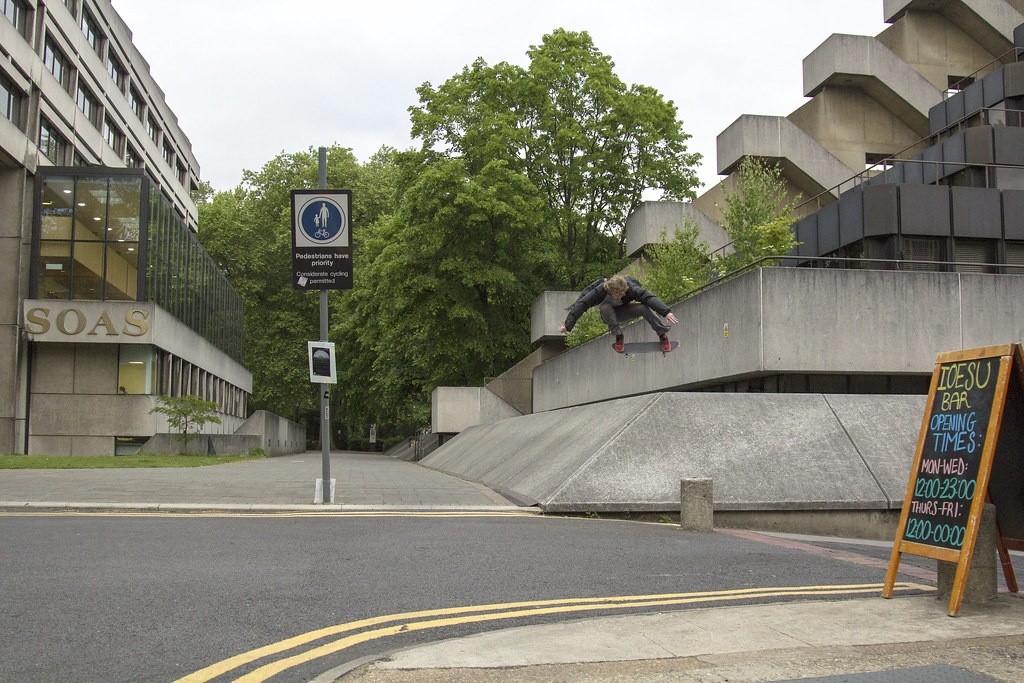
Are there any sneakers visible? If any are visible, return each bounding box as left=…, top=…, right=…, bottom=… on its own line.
left=659, top=333, right=671, bottom=352
left=615, top=332, right=625, bottom=353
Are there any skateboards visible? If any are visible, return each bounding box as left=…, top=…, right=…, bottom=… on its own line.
left=612, top=341, right=681, bottom=360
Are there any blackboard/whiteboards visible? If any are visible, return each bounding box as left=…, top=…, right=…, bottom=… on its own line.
left=900, top=342, right=1024, bottom=563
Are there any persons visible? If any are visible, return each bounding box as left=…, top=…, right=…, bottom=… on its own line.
left=560, top=275, right=679, bottom=351
